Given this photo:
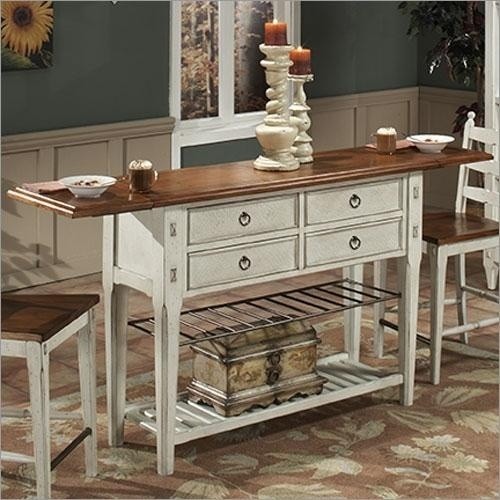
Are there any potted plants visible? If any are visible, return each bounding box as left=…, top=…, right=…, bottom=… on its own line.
left=398, top=0, right=485, bottom=220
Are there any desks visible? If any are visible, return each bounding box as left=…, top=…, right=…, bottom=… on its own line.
left=2, top=138, right=499, bottom=479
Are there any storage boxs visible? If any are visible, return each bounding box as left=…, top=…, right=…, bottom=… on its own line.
left=185, top=314, right=328, bottom=416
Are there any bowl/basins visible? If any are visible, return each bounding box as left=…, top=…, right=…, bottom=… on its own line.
left=405, top=134, right=455, bottom=153
left=59, top=176, right=117, bottom=199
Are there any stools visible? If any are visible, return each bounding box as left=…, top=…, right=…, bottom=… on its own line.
left=1, top=289, right=101, bottom=498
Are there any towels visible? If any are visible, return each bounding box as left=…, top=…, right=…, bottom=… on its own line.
left=22, top=180, right=68, bottom=194
left=365, top=139, right=415, bottom=150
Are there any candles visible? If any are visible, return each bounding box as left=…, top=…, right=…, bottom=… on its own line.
left=263, top=20, right=288, bottom=45
left=288, top=48, right=312, bottom=75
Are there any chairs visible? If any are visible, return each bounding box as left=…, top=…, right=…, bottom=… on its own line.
left=372, top=111, right=500, bottom=388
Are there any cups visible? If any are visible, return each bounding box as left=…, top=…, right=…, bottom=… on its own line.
left=372, top=133, right=397, bottom=155
left=128, top=167, right=158, bottom=193
